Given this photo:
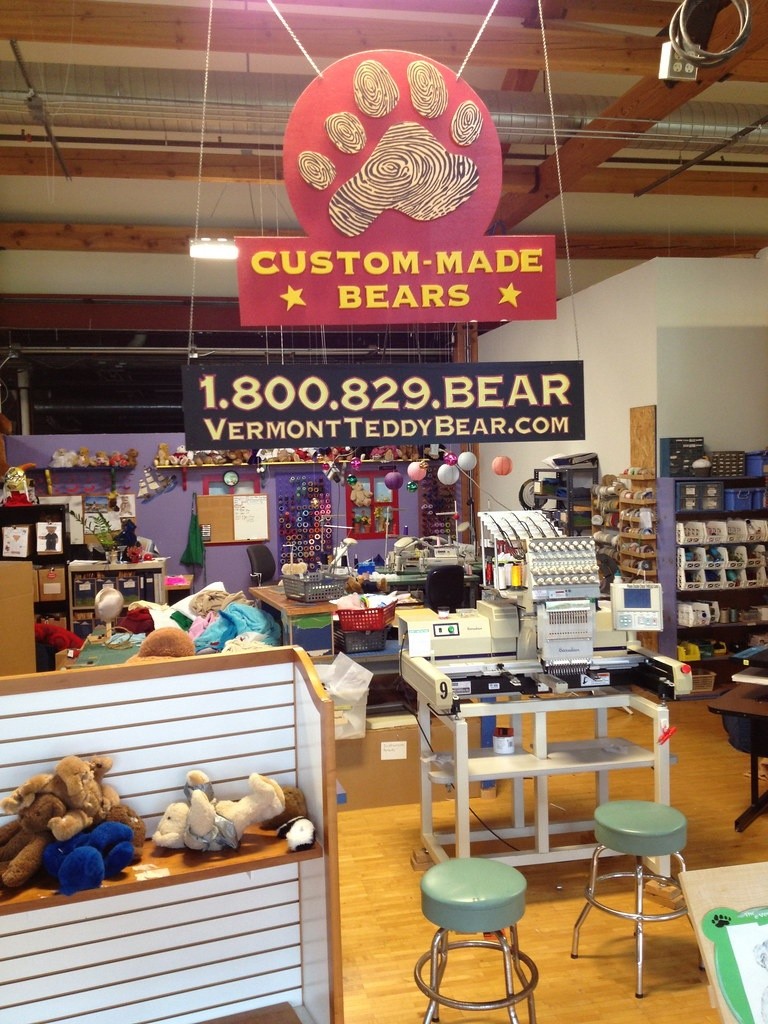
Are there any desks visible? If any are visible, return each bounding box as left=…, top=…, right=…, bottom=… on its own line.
left=167, top=574, right=194, bottom=598
left=248, top=582, right=421, bottom=724
left=709, top=667, right=768, bottom=833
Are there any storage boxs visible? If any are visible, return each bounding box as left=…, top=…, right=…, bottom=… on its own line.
left=73, top=574, right=139, bottom=635
left=746, top=451, right=768, bottom=476
left=31, top=566, right=41, bottom=603
left=675, top=482, right=767, bottom=694
left=38, top=563, right=68, bottom=603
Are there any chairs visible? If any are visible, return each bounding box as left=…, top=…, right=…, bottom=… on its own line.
left=246, top=542, right=278, bottom=587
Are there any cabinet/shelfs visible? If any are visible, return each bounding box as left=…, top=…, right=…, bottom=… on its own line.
left=531, top=464, right=660, bottom=587
left=0, top=503, right=72, bottom=633
left=67, top=559, right=170, bottom=638
left=657, top=476, right=768, bottom=700
left=415, top=681, right=673, bottom=891
left=0, top=643, right=345, bottom=1024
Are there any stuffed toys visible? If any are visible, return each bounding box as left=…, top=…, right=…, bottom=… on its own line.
left=276, top=815, right=316, bottom=853
left=260, top=786, right=308, bottom=827
left=350, top=482, right=373, bottom=506
left=9, top=535, right=23, bottom=554
left=153, top=443, right=446, bottom=467
left=0, top=750, right=148, bottom=902
left=345, top=576, right=391, bottom=593
left=49, top=448, right=139, bottom=467
left=151, top=769, right=287, bottom=852
left=39, top=526, right=58, bottom=552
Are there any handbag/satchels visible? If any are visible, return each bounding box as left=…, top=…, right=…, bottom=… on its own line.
left=180, top=491, right=205, bottom=566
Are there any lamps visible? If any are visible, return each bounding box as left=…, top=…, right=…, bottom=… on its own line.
left=326, top=536, right=359, bottom=574
left=394, top=536, right=438, bottom=553
left=457, top=523, right=477, bottom=546
left=392, top=535, right=448, bottom=572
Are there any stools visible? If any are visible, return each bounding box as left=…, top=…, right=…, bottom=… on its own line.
left=572, top=797, right=706, bottom=1001
left=407, top=855, right=542, bottom=1024
